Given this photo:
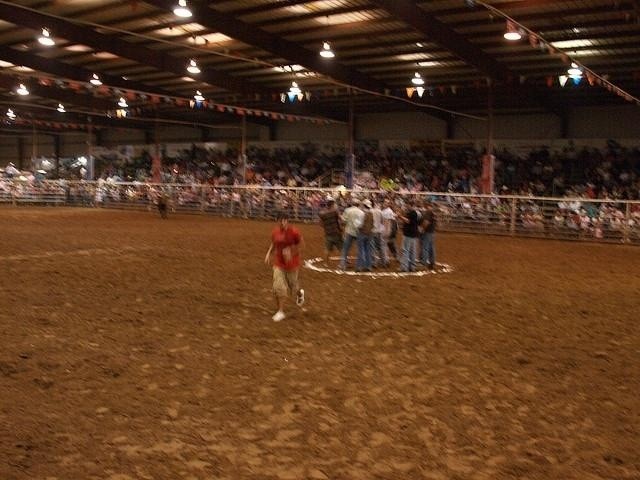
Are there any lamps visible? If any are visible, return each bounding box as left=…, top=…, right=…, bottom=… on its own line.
left=402, top=38, right=429, bottom=103
left=502, top=31, right=582, bottom=92
left=273, top=39, right=341, bottom=108
left=1, top=28, right=130, bottom=119
left=172, top=1, right=208, bottom=109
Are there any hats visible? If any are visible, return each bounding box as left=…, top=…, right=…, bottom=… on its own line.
left=352, top=198, right=360, bottom=204
left=326, top=196, right=334, bottom=201
left=363, top=198, right=373, bottom=208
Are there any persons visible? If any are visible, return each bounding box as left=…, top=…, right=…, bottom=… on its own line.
left=1, top=138, right=639, bottom=243
left=264, top=212, right=306, bottom=322
left=319, top=196, right=439, bottom=272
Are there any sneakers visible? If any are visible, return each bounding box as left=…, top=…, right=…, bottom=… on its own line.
left=325, top=262, right=331, bottom=267
left=384, top=254, right=416, bottom=272
left=272, top=311, right=286, bottom=322
left=296, top=288, right=305, bottom=306
left=418, top=257, right=435, bottom=267
left=339, top=264, right=377, bottom=272
